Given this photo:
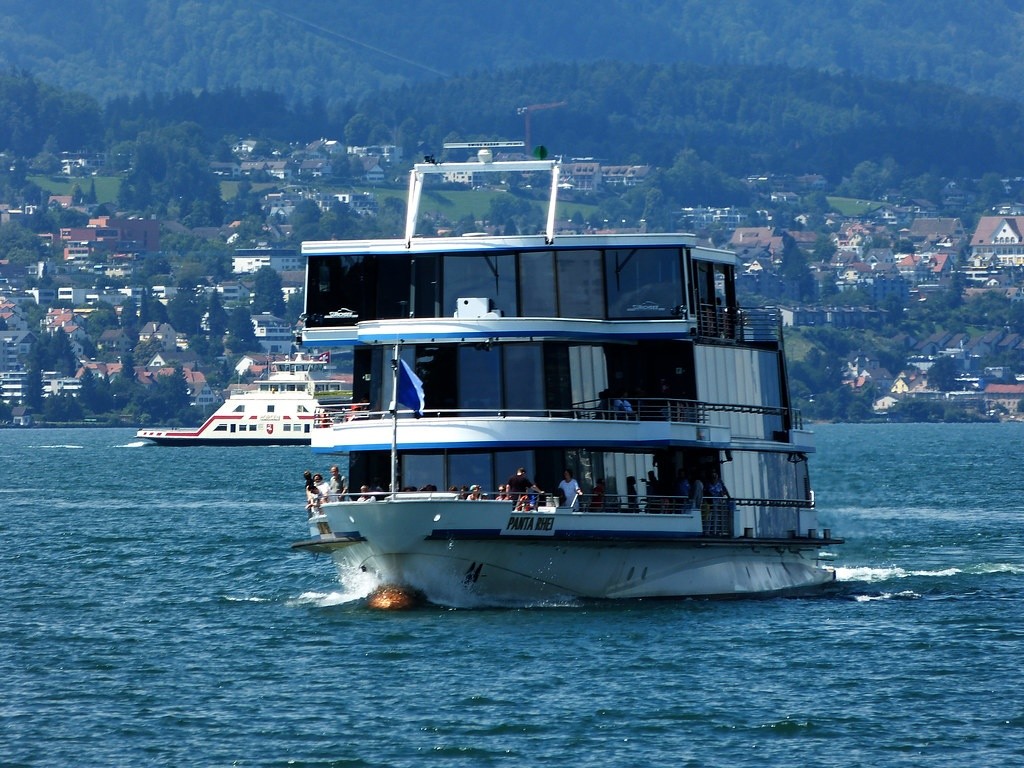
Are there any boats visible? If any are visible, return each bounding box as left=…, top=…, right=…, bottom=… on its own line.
left=290, top=155, right=856, bottom=605
left=135, top=388, right=327, bottom=446
left=245, top=352, right=315, bottom=398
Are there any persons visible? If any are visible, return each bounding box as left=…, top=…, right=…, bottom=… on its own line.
left=593, top=388, right=636, bottom=420
left=356, top=485, right=376, bottom=501
left=556, top=468, right=583, bottom=512
left=494, top=485, right=510, bottom=500
left=460, top=486, right=469, bottom=499
left=465, top=485, right=482, bottom=500
left=627, top=476, right=640, bottom=513
left=642, top=467, right=730, bottom=509
left=303, top=471, right=327, bottom=506
left=481, top=494, right=488, bottom=499
left=716, top=296, right=724, bottom=337
left=505, top=468, right=545, bottom=510
left=588, top=478, right=606, bottom=512
left=400, top=484, right=458, bottom=492
left=329, top=465, right=349, bottom=501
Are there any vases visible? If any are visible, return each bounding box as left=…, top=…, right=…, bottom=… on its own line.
left=546, top=496, right=560, bottom=508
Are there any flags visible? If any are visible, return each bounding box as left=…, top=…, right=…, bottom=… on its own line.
left=318, top=351, right=331, bottom=364
left=392, top=356, right=425, bottom=416
